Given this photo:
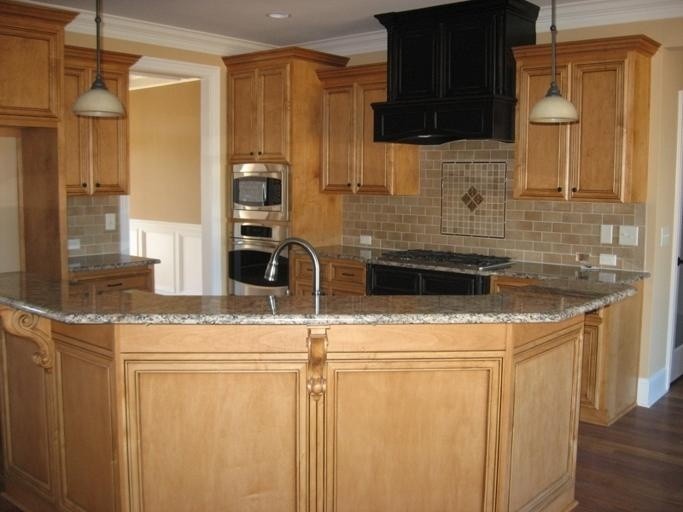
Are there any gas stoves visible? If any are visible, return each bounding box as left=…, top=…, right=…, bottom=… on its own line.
left=379, top=248, right=518, bottom=273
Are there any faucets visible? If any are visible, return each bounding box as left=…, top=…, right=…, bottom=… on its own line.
left=264, top=237, right=323, bottom=296
left=268, top=297, right=322, bottom=315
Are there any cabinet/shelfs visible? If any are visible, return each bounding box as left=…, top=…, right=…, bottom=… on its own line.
left=509, top=32, right=662, bottom=204
left=0, top=0, right=79, bottom=129
left=312, top=63, right=419, bottom=196
left=489, top=275, right=644, bottom=427
left=221, top=46, right=349, bottom=166
left=67, top=253, right=161, bottom=293
left=128, top=220, right=201, bottom=297
left=289, top=243, right=367, bottom=295
left=365, top=262, right=487, bottom=298
left=62, top=44, right=143, bottom=197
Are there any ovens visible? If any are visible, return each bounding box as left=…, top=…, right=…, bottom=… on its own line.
left=224, top=162, right=290, bottom=294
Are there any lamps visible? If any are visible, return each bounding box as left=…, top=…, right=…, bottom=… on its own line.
left=528, top=1, right=579, bottom=124
left=71, top=0, right=128, bottom=118
